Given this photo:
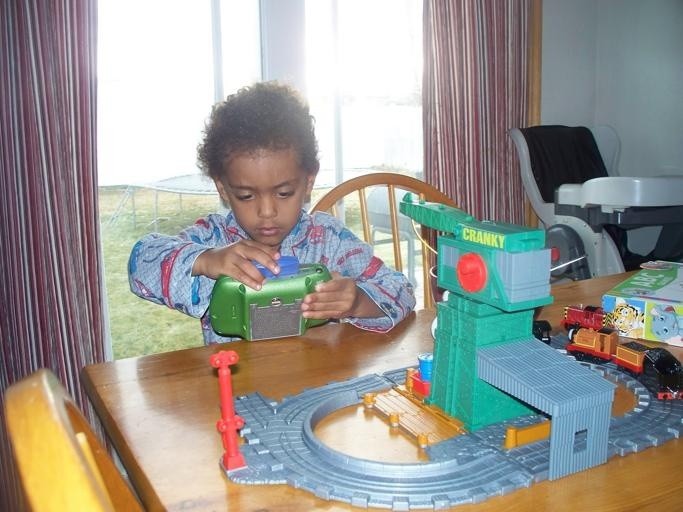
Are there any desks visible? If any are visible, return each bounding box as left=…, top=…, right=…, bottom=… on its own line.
left=79, top=266, right=683, bottom=512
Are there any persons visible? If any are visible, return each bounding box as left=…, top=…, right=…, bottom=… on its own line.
left=126, top=82, right=416, bottom=350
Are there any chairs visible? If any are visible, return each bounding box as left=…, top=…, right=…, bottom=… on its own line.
left=506, top=122, right=683, bottom=285
left=309, top=172, right=460, bottom=310
left=2, top=366, right=151, bottom=512
